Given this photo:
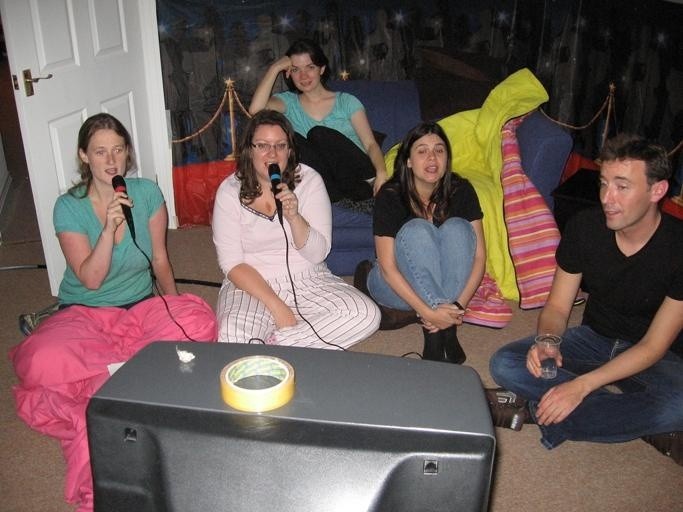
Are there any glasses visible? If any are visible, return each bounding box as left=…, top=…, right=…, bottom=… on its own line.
left=249, top=141, right=286, bottom=154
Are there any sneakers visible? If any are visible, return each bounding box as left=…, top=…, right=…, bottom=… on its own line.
left=19, top=305, right=60, bottom=335
left=484, top=386, right=531, bottom=431
left=641, top=429, right=682, bottom=466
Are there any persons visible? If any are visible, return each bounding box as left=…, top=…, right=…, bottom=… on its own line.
left=484, top=132, right=683, bottom=450
left=367, top=120, right=487, bottom=365
left=212, top=109, right=382, bottom=350
left=8, top=112, right=217, bottom=512
left=248, top=41, right=389, bottom=203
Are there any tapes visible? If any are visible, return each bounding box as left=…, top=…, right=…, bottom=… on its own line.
left=220, top=353, right=295, bottom=414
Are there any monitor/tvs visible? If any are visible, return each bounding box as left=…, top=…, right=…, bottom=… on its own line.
left=84, top=340, right=498, bottom=512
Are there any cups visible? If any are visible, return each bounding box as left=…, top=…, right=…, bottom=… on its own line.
left=534, top=334, right=562, bottom=381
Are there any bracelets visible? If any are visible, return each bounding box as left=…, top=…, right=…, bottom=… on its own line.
left=453, top=299, right=465, bottom=311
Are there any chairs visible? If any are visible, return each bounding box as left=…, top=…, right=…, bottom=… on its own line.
left=514, top=110, right=574, bottom=218
left=303, top=80, right=426, bottom=274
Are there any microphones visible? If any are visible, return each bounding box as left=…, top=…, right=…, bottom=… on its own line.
left=112, top=175, right=135, bottom=241
left=268, top=163, right=283, bottom=225
